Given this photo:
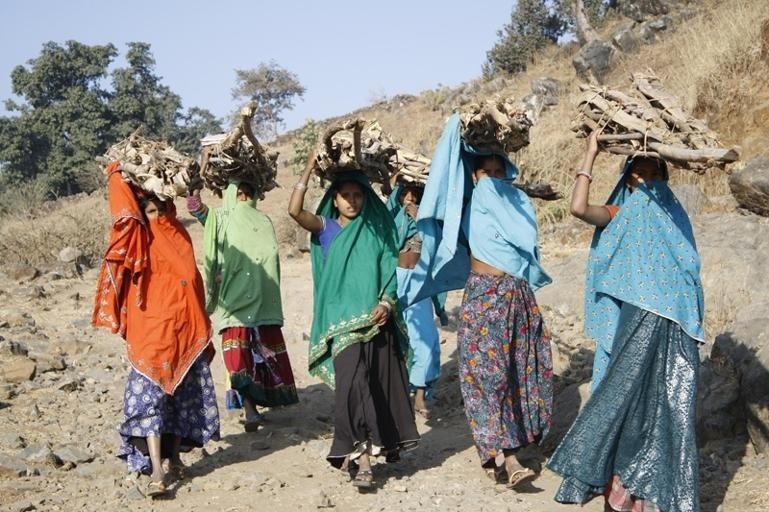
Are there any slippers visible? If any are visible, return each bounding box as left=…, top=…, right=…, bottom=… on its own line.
left=169, top=460, right=186, bottom=480
left=494, top=463, right=510, bottom=484
left=353, top=468, right=373, bottom=489
left=146, top=475, right=168, bottom=494
left=506, top=465, right=537, bottom=489
left=414, top=404, right=436, bottom=421
left=244, top=412, right=261, bottom=432
left=386, top=443, right=401, bottom=463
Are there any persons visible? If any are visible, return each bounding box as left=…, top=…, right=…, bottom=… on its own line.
left=399, top=112, right=554, bottom=490
left=288, top=144, right=449, bottom=490
left=543, top=128, right=706, bottom=512
left=187, top=179, right=300, bottom=432
left=91, top=158, right=220, bottom=497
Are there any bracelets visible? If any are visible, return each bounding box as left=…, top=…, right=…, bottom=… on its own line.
left=575, top=171, right=593, bottom=183
